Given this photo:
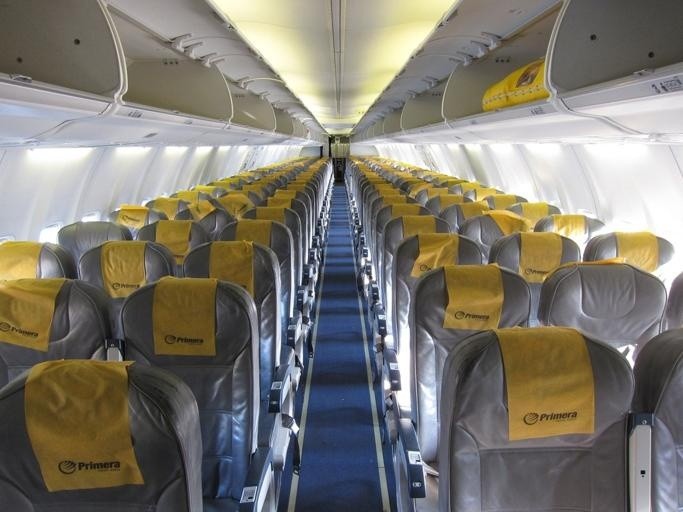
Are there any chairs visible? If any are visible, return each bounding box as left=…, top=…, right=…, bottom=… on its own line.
left=1, top=154, right=336, bottom=511
left=344, top=154, right=680, bottom=510
